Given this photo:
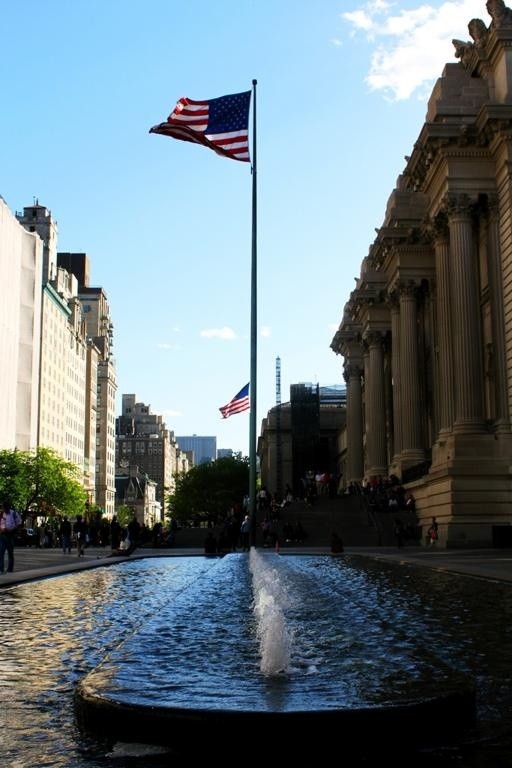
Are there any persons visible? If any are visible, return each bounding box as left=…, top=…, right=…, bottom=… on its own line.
left=0, top=501, right=22, bottom=572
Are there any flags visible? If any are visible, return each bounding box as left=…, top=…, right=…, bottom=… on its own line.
left=220, top=383, right=250, bottom=418
left=150, top=90, right=250, bottom=161
